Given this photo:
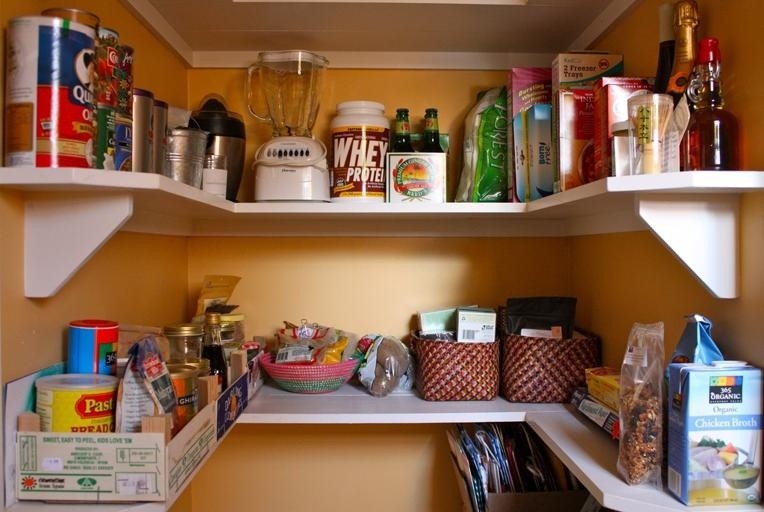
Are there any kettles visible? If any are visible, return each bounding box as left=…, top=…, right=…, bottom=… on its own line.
left=189, top=96, right=244, bottom=202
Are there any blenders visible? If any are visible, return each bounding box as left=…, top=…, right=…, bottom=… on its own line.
left=246, top=48, right=332, bottom=203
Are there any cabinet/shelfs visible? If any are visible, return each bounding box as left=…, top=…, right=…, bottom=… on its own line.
left=0, top=164, right=764, bottom=512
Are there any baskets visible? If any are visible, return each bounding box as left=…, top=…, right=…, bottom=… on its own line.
left=503, top=330, right=601, bottom=403
left=411, top=329, right=499, bottom=401
left=260, top=352, right=364, bottom=395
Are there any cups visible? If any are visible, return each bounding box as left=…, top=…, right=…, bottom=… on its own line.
left=204, top=154, right=226, bottom=170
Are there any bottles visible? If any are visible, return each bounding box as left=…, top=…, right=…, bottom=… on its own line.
left=153, top=96, right=166, bottom=173
left=161, top=320, right=202, bottom=369
left=395, top=108, right=412, bottom=151
left=626, top=93, right=674, bottom=173
left=329, top=97, right=388, bottom=200
left=130, top=86, right=151, bottom=173
left=420, top=108, right=443, bottom=152
left=675, top=39, right=740, bottom=170
left=668, top=2, right=702, bottom=95
left=653, top=2, right=675, bottom=93
left=200, top=311, right=227, bottom=391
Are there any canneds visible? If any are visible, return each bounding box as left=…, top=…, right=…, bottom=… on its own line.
left=96, top=25, right=121, bottom=108
left=113, top=112, right=137, bottom=172
left=120, top=44, right=137, bottom=120
left=32, top=372, right=122, bottom=433
left=163, top=362, right=201, bottom=422
left=6, top=16, right=96, bottom=170
left=96, top=102, right=116, bottom=169
